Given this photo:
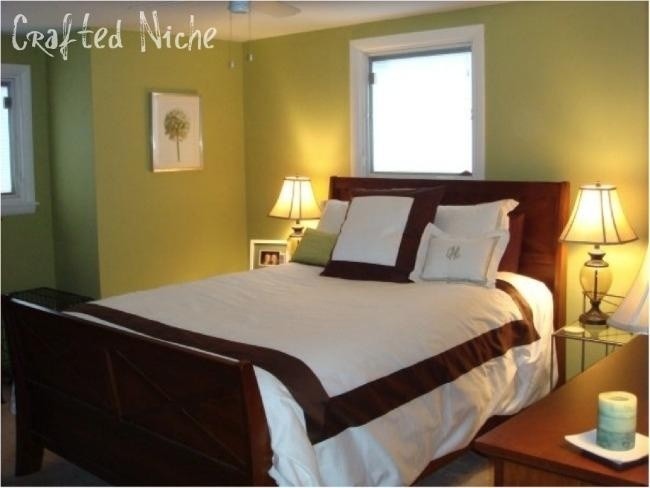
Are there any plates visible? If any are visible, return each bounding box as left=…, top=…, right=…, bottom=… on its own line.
left=565, top=427, right=649, bottom=465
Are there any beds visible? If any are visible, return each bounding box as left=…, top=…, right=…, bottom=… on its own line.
left=1, top=176, right=574, bottom=487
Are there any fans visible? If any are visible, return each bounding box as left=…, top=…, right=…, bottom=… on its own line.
left=223, top=1, right=300, bottom=21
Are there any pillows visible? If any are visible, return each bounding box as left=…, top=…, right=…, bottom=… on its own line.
left=434, top=200, right=520, bottom=236
left=316, top=198, right=349, bottom=234
left=288, top=227, right=339, bottom=265
left=330, top=194, right=416, bottom=266
left=408, top=222, right=509, bottom=291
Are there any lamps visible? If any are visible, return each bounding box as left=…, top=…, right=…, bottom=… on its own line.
left=266, top=175, right=323, bottom=257
left=559, top=181, right=640, bottom=325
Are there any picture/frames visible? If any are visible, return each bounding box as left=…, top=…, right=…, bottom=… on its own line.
left=149, top=89, right=203, bottom=174
left=249, top=240, right=288, bottom=270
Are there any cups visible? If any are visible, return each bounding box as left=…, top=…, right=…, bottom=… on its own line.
left=596, top=391, right=637, bottom=450
